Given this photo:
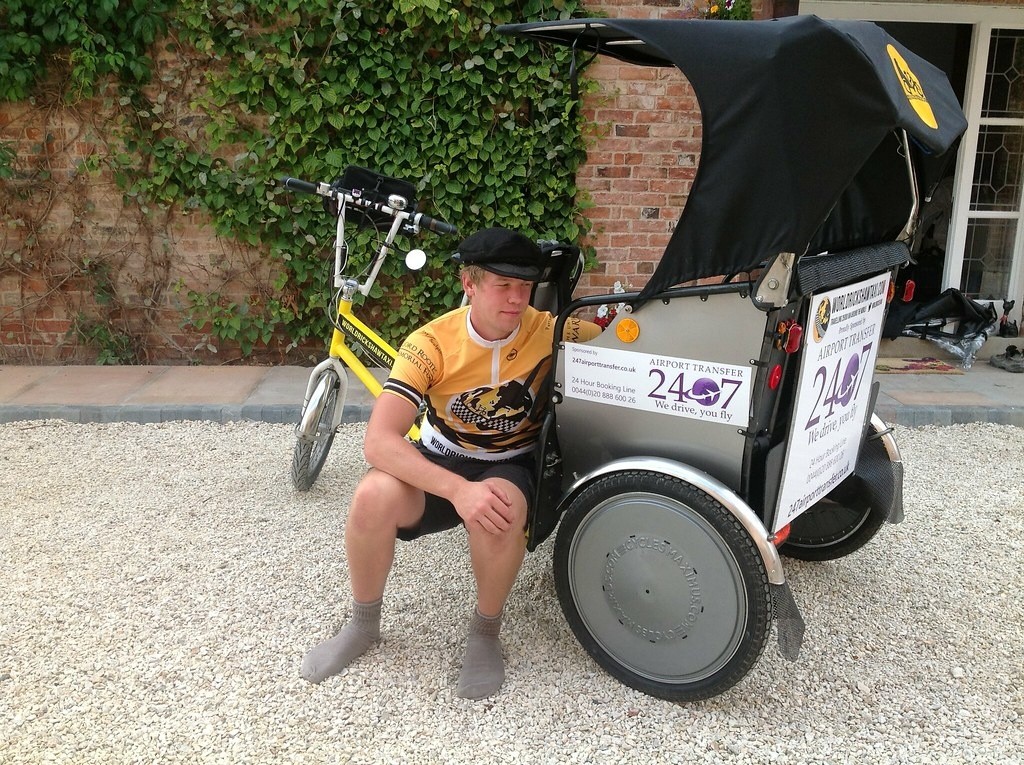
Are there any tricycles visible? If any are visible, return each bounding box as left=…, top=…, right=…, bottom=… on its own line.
left=288, top=15, right=969, bottom=704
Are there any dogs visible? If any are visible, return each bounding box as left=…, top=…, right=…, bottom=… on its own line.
left=995, top=298, right=1018, bottom=338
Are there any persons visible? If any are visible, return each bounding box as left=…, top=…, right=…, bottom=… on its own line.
left=294, top=222, right=608, bottom=703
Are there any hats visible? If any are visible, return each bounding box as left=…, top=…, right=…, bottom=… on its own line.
left=456, top=226, right=543, bottom=280
